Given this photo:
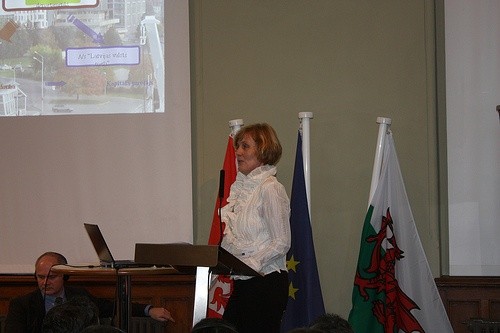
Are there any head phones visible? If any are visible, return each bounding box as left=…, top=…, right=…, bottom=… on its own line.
left=35, top=253, right=70, bottom=281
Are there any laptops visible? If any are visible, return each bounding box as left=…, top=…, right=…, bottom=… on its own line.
left=83, top=223, right=154, bottom=268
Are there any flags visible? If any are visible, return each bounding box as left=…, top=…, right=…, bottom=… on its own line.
left=205, top=134, right=239, bottom=319
left=286, top=127, right=326, bottom=330
left=346, top=130, right=454, bottom=333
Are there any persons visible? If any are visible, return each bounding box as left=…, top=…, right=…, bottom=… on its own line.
left=288, top=311, right=355, bottom=333
left=217, top=122, right=291, bottom=333
left=191, top=318, right=238, bottom=333
left=5, top=252, right=176, bottom=333
left=41, top=298, right=100, bottom=333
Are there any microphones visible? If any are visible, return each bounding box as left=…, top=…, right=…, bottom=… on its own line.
left=218, top=169, right=225, bottom=245
